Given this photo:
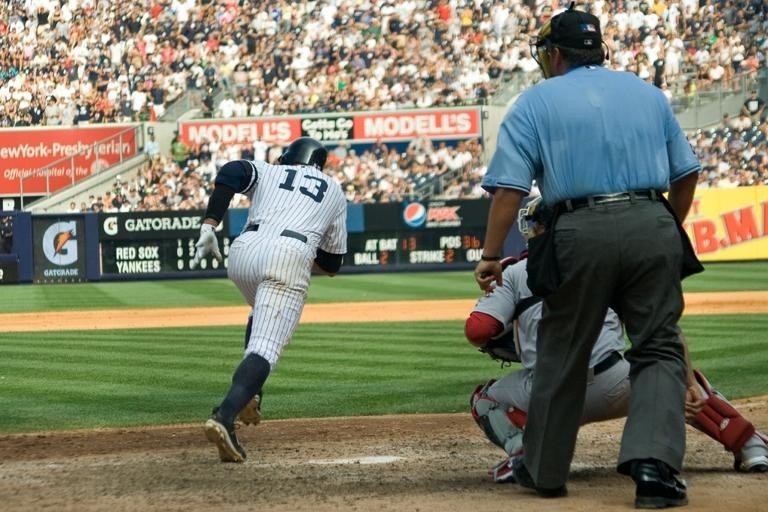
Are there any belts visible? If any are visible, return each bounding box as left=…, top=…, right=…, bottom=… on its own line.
left=245, top=224, right=307, bottom=245
left=549, top=189, right=658, bottom=208
left=593, top=353, right=620, bottom=375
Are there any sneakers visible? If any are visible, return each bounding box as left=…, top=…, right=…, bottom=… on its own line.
left=513, top=458, right=568, bottom=497
left=494, top=453, right=523, bottom=484
left=204, top=411, right=246, bottom=462
left=628, top=462, right=689, bottom=507
left=240, top=393, right=262, bottom=426
left=734, top=436, right=767, bottom=472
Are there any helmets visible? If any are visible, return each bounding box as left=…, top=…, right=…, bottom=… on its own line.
left=516, top=197, right=556, bottom=240
left=278, top=138, right=325, bottom=167
left=530, top=8, right=606, bottom=69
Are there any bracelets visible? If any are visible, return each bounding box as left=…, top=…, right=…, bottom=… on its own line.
left=480, top=254, right=501, bottom=261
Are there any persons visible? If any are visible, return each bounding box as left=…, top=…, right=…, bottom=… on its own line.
left=325, top=131, right=488, bottom=202
left=69, top=129, right=288, bottom=213
left=572, top=0, right=768, bottom=185
left=471, top=8, right=701, bottom=509
left=194, top=137, right=348, bottom=464
left=1, top=1, right=565, bottom=126
left=463, top=196, right=767, bottom=484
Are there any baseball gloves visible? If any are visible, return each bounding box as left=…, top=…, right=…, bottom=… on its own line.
left=485, top=330, right=519, bottom=362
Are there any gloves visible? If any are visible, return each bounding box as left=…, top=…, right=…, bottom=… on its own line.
left=192, top=223, right=222, bottom=265
